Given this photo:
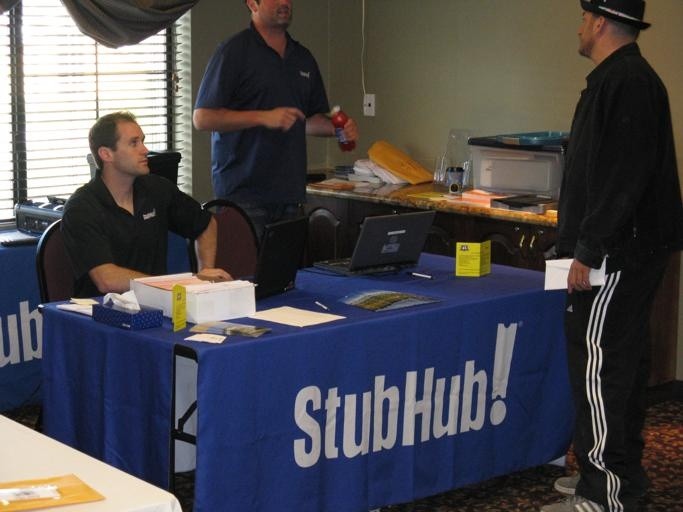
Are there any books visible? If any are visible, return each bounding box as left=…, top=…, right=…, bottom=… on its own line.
left=337, top=288, right=443, bottom=314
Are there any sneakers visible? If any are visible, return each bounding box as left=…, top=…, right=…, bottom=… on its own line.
left=540, top=494, right=607, bottom=511
left=555, top=473, right=581, bottom=494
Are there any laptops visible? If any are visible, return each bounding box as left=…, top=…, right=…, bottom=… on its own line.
left=242, top=216, right=310, bottom=299
left=314, top=210, right=436, bottom=276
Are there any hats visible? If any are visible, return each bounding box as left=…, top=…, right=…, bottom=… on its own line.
left=580, top=0, right=651, bottom=29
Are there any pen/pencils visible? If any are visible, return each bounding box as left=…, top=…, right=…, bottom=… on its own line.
left=315, top=301, right=330, bottom=312
left=406, top=271, right=434, bottom=280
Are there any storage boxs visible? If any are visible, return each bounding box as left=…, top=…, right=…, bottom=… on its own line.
left=468, top=132, right=572, bottom=196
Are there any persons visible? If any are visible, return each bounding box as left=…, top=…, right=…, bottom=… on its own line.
left=59, top=111, right=235, bottom=300
left=190, top=0, right=358, bottom=245
left=537, top=0, right=680, bottom=511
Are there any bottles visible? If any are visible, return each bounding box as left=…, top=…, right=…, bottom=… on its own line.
left=330, top=104, right=357, bottom=152
left=445, top=166, right=464, bottom=195
